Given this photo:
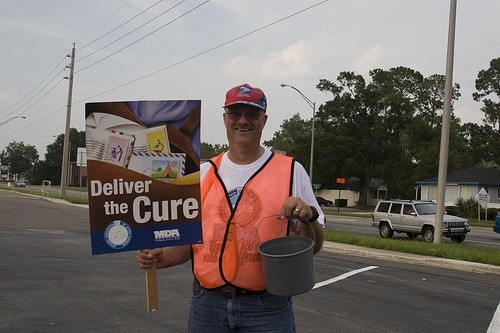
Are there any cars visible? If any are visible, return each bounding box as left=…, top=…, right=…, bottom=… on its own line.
left=14, top=180, right=25, bottom=187
left=315, top=196, right=333, bottom=207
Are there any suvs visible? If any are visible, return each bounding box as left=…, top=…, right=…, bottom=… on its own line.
left=371, top=198, right=471, bottom=242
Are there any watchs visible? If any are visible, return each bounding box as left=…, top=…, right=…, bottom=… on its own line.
left=300, top=205, right=319, bottom=224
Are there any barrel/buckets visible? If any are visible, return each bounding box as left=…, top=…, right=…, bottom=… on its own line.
left=254, top=213, right=316, bottom=296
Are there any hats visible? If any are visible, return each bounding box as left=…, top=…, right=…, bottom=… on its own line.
left=221, top=83, right=268, bottom=112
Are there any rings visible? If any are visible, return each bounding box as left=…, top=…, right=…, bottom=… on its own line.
left=295, top=208, right=301, bottom=213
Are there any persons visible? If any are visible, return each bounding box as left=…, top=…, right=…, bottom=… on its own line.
left=136, top=84, right=325, bottom=333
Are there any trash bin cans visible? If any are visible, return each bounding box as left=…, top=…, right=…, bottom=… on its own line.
left=335, top=199, right=348, bottom=207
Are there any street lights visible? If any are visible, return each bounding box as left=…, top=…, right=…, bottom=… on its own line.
left=280, top=83, right=316, bottom=184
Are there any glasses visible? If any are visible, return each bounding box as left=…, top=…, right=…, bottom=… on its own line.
left=225, top=110, right=261, bottom=118
